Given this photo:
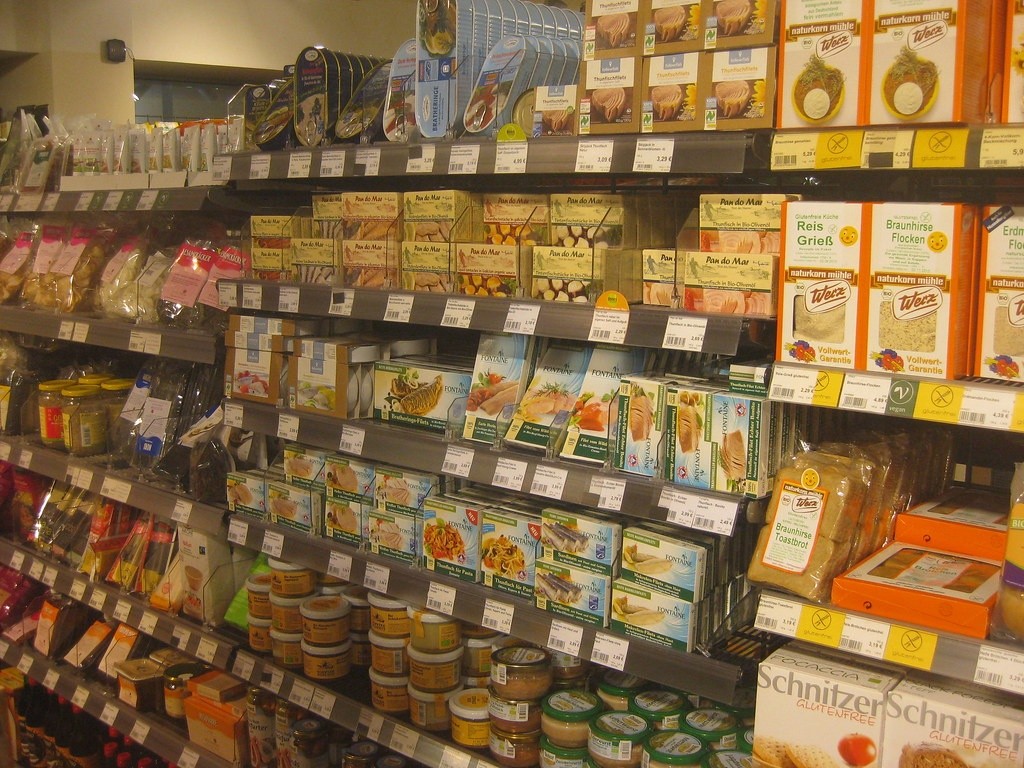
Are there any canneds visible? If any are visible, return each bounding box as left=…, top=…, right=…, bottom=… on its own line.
left=242, top=0, right=585, bottom=154
left=245, top=556, right=755, bottom=768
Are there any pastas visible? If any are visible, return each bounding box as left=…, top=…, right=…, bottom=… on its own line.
left=424, top=523, right=466, bottom=560
left=482, top=535, right=526, bottom=580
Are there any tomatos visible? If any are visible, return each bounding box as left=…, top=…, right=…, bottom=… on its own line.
left=838, top=733, right=877, bottom=765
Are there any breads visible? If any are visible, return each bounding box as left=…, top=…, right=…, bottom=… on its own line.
left=746, top=415, right=967, bottom=605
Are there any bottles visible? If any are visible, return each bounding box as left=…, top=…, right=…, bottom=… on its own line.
left=37, top=370, right=77, bottom=450
left=246, top=686, right=407, bottom=768
left=17, top=671, right=178, bottom=768
left=77, top=372, right=114, bottom=393
left=102, top=379, right=133, bottom=452
left=58, top=383, right=106, bottom=457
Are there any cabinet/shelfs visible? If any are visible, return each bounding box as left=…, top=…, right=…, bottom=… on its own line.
left=0, top=120, right=1024, bottom=768
